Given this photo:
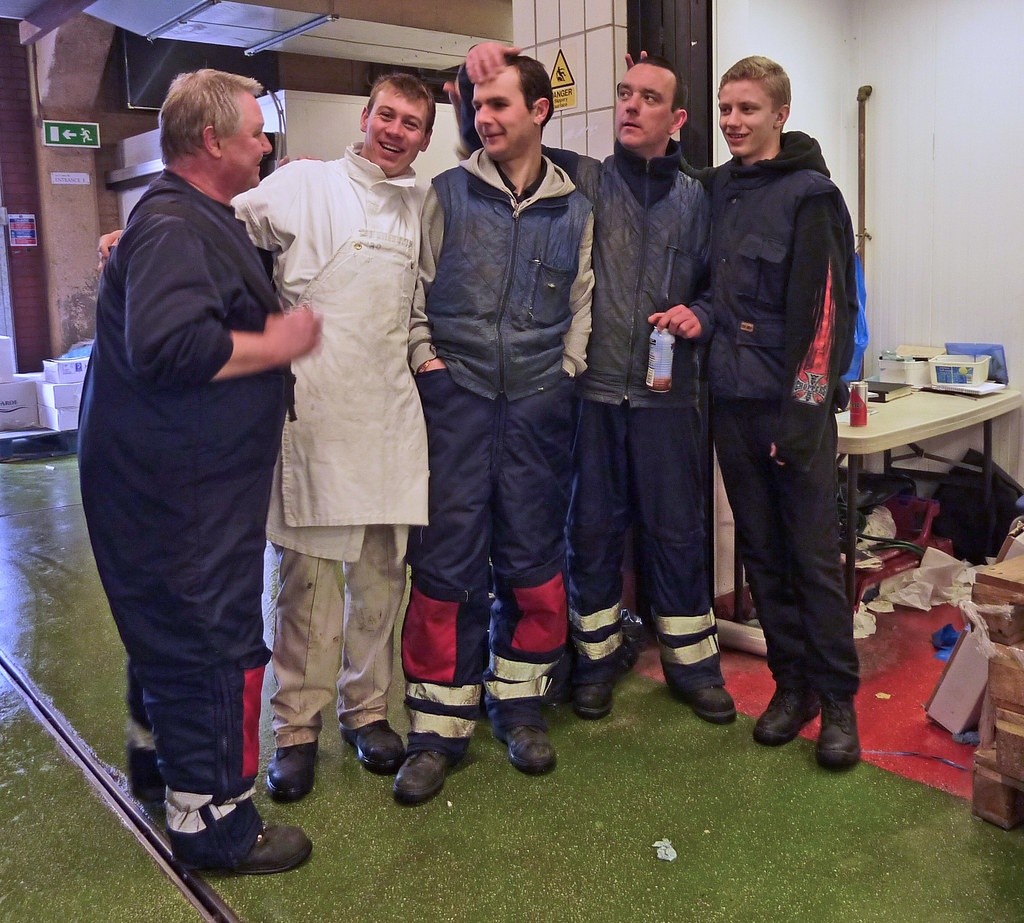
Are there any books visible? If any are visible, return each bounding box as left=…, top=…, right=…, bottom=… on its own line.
left=932, top=382, right=1004, bottom=396
left=862, top=381, right=914, bottom=404
left=878, top=344, right=945, bottom=361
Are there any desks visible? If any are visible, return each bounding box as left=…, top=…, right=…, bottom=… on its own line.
left=728, top=386, right=1024, bottom=623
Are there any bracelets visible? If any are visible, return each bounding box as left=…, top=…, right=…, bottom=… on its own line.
left=419, top=359, right=431, bottom=374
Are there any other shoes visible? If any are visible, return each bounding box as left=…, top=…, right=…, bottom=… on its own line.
left=668, top=684, right=737, bottom=725
left=231, top=820, right=311, bottom=873
left=127, top=739, right=166, bottom=803
left=570, top=682, right=615, bottom=718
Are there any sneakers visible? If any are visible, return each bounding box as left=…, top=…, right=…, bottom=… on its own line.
left=267, top=738, right=319, bottom=800
left=493, top=725, right=558, bottom=773
left=392, top=749, right=457, bottom=803
left=752, top=680, right=820, bottom=746
left=814, top=684, right=860, bottom=772
left=340, top=720, right=406, bottom=775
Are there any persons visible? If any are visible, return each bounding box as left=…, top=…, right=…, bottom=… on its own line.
left=625, top=48, right=860, bottom=768
left=98, top=73, right=462, bottom=804
left=76, top=70, right=320, bottom=875
left=392, top=56, right=595, bottom=802
left=457, top=41, right=738, bottom=724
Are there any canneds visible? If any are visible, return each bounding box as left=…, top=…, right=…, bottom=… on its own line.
left=849, top=381, right=869, bottom=428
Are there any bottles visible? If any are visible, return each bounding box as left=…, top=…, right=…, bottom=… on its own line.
left=645, top=317, right=676, bottom=393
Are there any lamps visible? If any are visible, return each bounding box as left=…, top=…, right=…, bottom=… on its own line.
left=244, top=14, right=339, bottom=57
left=146, top=0, right=221, bottom=42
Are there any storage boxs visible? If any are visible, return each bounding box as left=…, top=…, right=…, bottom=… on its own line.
left=925, top=523, right=1024, bottom=736
left=928, top=355, right=991, bottom=386
left=0, top=335, right=91, bottom=432
